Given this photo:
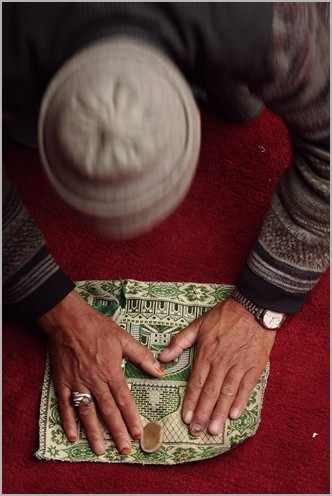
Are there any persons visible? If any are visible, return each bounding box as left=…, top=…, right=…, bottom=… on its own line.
left=2, top=2, right=331, bottom=455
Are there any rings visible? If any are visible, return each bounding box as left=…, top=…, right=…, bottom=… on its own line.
left=71, top=390, right=93, bottom=408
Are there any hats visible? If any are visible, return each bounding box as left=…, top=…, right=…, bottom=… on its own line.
left=39, top=37, right=202, bottom=238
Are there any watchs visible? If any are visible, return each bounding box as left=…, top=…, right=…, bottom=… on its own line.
left=230, top=289, right=287, bottom=330
